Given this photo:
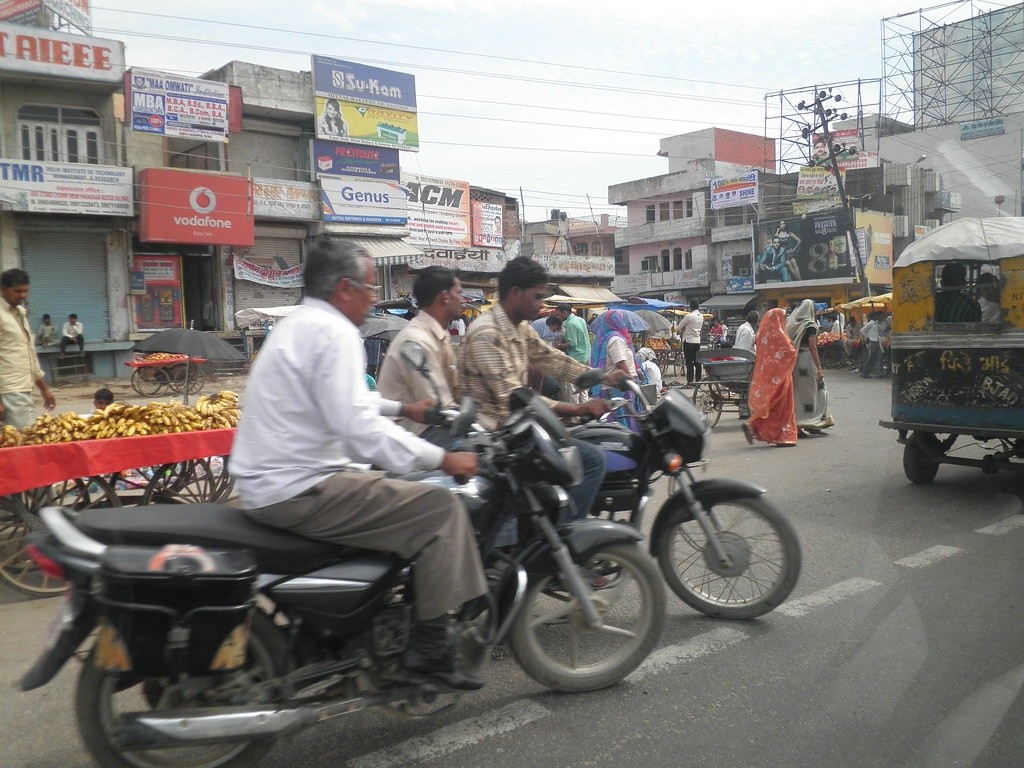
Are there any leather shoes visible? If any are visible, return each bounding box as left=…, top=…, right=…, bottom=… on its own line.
left=387, top=649, right=486, bottom=693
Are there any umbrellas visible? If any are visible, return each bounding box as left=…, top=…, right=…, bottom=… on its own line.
left=618, top=309, right=650, bottom=333
left=633, top=310, right=671, bottom=336
left=129, top=319, right=247, bottom=404
left=824, top=292, right=892, bottom=325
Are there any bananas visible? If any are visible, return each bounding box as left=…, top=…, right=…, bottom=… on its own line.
left=0, top=388, right=243, bottom=448
left=818, top=331, right=847, bottom=344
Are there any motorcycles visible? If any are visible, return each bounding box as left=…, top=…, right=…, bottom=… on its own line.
left=707, top=334, right=721, bottom=350
left=19, top=367, right=803, bottom=768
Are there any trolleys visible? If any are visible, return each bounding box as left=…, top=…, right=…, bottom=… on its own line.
left=125, top=357, right=210, bottom=397
left=0, top=427, right=240, bottom=597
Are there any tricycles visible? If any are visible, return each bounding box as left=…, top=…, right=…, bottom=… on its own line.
left=879, top=217, right=1024, bottom=485
left=814, top=292, right=893, bottom=376
left=689, top=341, right=760, bottom=429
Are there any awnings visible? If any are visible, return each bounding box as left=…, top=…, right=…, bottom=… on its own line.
left=558, top=285, right=625, bottom=303
left=698, top=295, right=759, bottom=310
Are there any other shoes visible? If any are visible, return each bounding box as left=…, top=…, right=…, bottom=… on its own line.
left=679, top=384, right=694, bottom=389
left=798, top=429, right=829, bottom=439
left=777, top=441, right=798, bottom=447
left=58, top=353, right=65, bottom=359
left=740, top=422, right=753, bottom=445
left=80, top=352, right=85, bottom=357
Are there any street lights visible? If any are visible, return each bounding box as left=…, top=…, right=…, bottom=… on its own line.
left=796, top=90, right=869, bottom=298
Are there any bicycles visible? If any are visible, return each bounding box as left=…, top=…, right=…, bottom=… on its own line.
left=656, top=350, right=689, bottom=377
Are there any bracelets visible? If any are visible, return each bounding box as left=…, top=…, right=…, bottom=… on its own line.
left=558, top=343, right=562, bottom=349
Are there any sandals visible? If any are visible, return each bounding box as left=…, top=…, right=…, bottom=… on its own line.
left=545, top=567, right=610, bottom=591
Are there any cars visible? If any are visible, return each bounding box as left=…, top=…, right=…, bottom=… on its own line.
left=373, top=295, right=490, bottom=328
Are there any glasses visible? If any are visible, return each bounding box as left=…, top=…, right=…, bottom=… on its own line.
left=342, top=277, right=380, bottom=297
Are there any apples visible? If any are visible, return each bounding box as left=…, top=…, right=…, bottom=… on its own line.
left=650, top=338, right=667, bottom=350
left=142, top=352, right=185, bottom=363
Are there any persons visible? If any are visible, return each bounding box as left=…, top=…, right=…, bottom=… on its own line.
left=706, top=318, right=728, bottom=348
left=93, top=389, right=114, bottom=410
left=741, top=298, right=835, bottom=447
left=758, top=139, right=837, bottom=283
left=0, top=269, right=57, bottom=507
left=588, top=310, right=649, bottom=433
left=825, top=311, right=891, bottom=379
left=674, top=298, right=704, bottom=390
left=457, top=258, right=633, bottom=588
left=546, top=316, right=568, bottom=403
left=555, top=303, right=590, bottom=424
left=734, top=311, right=760, bottom=422
left=318, top=98, right=346, bottom=137
left=375, top=264, right=470, bottom=454
left=226, top=239, right=490, bottom=689
left=37, top=313, right=57, bottom=348
left=60, top=314, right=85, bottom=360
left=494, top=215, right=503, bottom=235
left=637, top=346, right=662, bottom=393
left=936, top=262, right=999, bottom=323
